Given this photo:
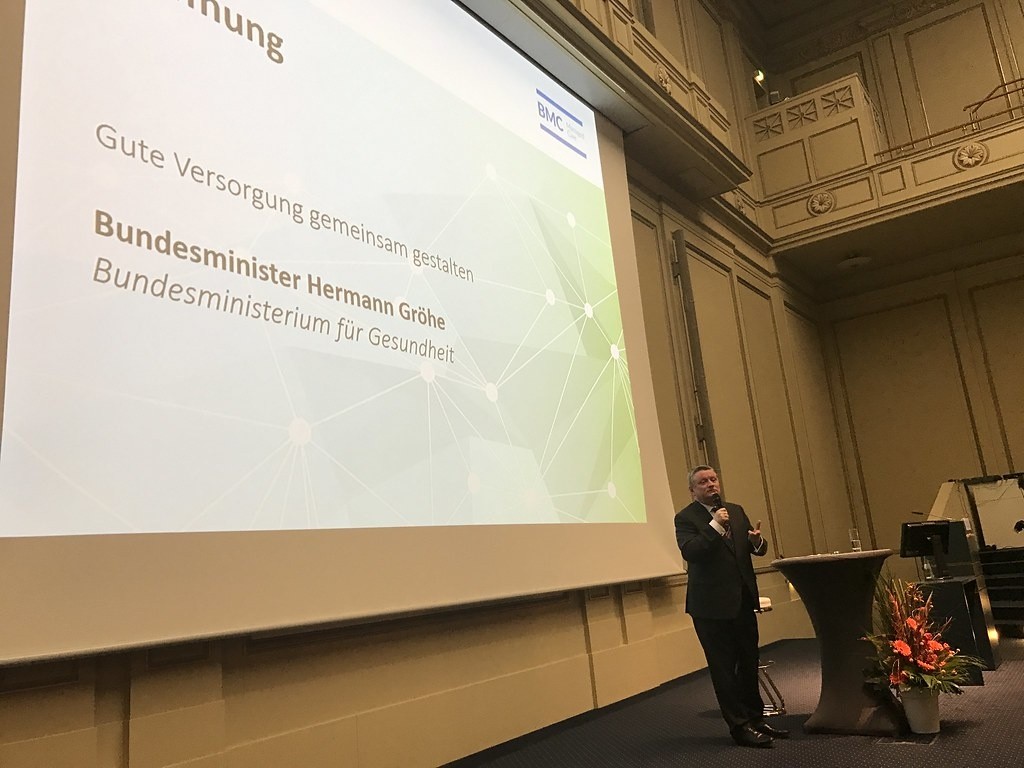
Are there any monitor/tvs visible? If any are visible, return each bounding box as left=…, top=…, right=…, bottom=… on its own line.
left=900, top=520, right=953, bottom=580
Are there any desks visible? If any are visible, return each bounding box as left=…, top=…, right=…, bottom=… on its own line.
left=772, top=548, right=911, bottom=735
left=915, top=574, right=996, bottom=686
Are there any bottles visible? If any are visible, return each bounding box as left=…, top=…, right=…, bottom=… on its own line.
left=923, top=556, right=933, bottom=579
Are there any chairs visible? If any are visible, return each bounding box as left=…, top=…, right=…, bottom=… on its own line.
left=756, top=597, right=787, bottom=717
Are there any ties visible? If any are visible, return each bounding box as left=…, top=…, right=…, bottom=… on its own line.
left=710, top=507, right=735, bottom=553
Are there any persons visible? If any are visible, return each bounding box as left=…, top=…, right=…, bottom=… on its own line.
left=677, top=459, right=797, bottom=745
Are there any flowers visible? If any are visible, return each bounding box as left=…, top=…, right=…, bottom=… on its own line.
left=856, top=560, right=989, bottom=697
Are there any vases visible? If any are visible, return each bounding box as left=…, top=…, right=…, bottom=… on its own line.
left=898, top=686, right=940, bottom=733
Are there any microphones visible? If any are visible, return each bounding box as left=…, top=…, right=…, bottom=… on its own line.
left=711, top=494, right=729, bottom=529
left=911, top=511, right=951, bottom=520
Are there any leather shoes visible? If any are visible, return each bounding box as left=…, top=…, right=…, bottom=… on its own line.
left=734, top=727, right=776, bottom=747
left=757, top=722, right=790, bottom=737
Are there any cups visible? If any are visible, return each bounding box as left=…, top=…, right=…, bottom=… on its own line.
left=848, top=528, right=862, bottom=552
